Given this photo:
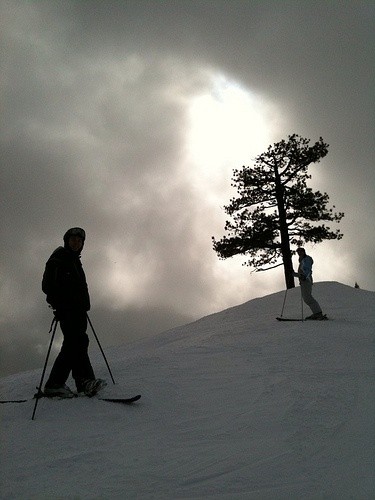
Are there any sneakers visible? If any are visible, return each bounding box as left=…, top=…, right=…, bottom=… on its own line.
left=43, top=384, right=78, bottom=399
left=83, top=377, right=107, bottom=396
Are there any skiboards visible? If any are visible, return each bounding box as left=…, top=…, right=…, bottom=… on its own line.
left=276, top=314, right=328, bottom=321
left=0, top=394, right=142, bottom=404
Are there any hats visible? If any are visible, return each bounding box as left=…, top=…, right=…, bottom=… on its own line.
left=64, top=227, right=86, bottom=240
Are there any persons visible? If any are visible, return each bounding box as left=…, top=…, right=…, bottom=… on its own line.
left=42, top=227, right=107, bottom=398
left=292, top=248, right=322, bottom=320
